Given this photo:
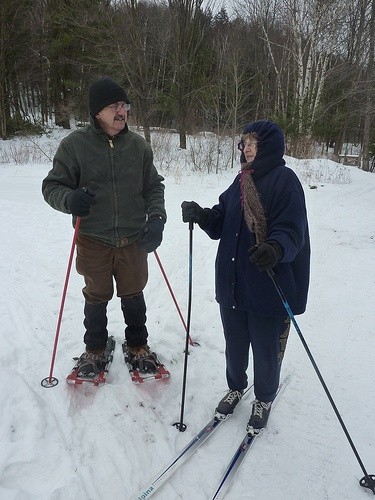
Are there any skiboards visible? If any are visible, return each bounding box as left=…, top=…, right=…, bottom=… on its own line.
left=208, top=366, right=295, bottom=500
left=121, top=340, right=171, bottom=385
left=66, top=334, right=116, bottom=387
left=135, top=382, right=254, bottom=500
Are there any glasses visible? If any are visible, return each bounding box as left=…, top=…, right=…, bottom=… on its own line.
left=105, top=103, right=131, bottom=111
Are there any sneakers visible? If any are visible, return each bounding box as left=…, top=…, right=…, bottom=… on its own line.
left=217, top=390, right=243, bottom=414
left=247, top=400, right=272, bottom=430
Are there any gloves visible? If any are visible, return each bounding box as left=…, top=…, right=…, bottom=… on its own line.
left=67, top=187, right=96, bottom=217
left=247, top=240, right=282, bottom=273
left=181, top=200, right=204, bottom=223
left=135, top=216, right=164, bottom=253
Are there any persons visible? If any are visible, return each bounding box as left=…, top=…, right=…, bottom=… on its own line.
left=41, top=78, right=168, bottom=382
left=181, top=120, right=311, bottom=436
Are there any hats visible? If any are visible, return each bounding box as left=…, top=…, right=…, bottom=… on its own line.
left=89, top=79, right=129, bottom=118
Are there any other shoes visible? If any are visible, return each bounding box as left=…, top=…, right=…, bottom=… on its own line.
left=78, top=350, right=106, bottom=377
left=128, top=345, right=157, bottom=374
